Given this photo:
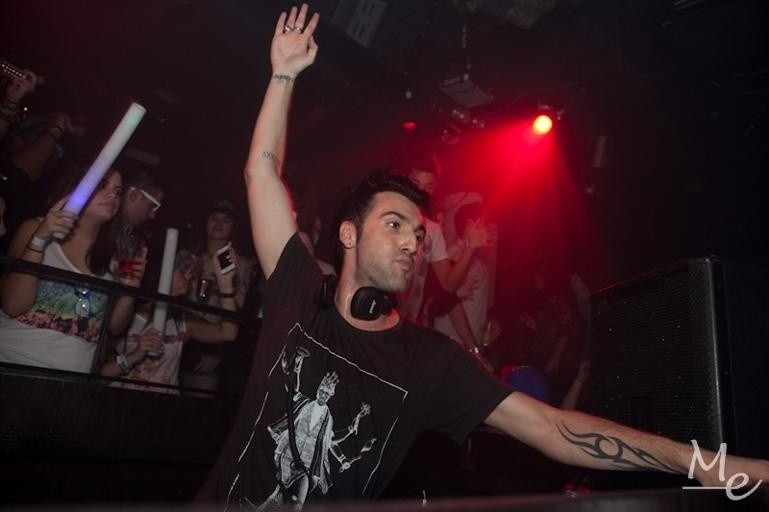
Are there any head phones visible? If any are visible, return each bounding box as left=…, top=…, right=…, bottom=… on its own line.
left=316, top=273, right=397, bottom=322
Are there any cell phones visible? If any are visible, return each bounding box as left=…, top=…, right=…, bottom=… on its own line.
left=215, top=242, right=235, bottom=275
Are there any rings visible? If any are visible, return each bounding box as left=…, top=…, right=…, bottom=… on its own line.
left=296, top=27, right=303, bottom=32
left=286, top=26, right=291, bottom=32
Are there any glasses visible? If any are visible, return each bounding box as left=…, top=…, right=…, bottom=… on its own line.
left=131, top=186, right=160, bottom=213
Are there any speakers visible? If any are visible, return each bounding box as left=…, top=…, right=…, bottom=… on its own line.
left=587, top=252, right=769, bottom=491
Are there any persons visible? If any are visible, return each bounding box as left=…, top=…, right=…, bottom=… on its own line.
left=269, top=351, right=352, bottom=512
left=181, top=5, right=769, bottom=512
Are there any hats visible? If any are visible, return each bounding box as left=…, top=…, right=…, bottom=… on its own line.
left=210, top=198, right=237, bottom=221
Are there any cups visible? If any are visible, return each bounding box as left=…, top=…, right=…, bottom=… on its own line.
left=116, top=233, right=146, bottom=280
